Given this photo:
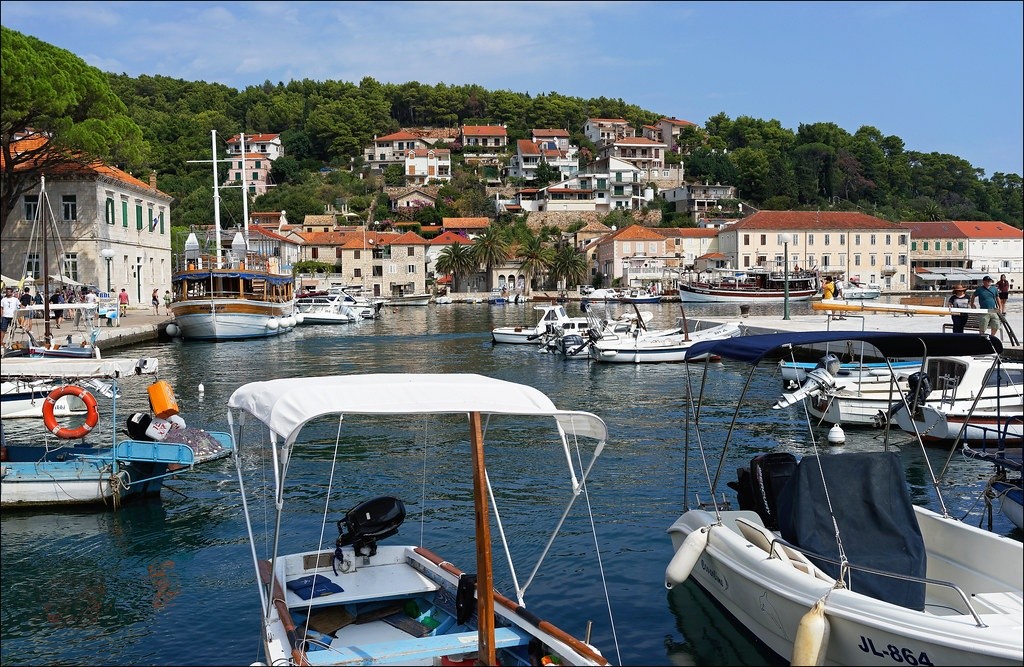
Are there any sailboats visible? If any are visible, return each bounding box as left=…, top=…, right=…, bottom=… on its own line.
left=170, top=130, right=300, bottom=339
left=0, top=173, right=159, bottom=381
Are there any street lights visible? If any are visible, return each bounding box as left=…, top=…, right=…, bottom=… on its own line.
left=344, top=212, right=370, bottom=296
left=780, top=234, right=792, bottom=322
left=100, top=248, right=115, bottom=327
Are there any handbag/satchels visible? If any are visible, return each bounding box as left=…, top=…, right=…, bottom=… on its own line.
left=58, top=295, right=65, bottom=304
left=822, top=294, right=825, bottom=299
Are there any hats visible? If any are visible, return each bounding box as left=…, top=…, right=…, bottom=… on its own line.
left=983, top=276, right=992, bottom=280
left=954, top=285, right=967, bottom=290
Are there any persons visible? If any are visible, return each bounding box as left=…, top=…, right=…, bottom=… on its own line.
left=648, top=284, right=657, bottom=294
left=948, top=274, right=1010, bottom=336
left=0, top=287, right=129, bottom=347
left=821, top=274, right=860, bottom=322
left=668, top=281, right=674, bottom=294
left=163, top=290, right=172, bottom=316
left=152, top=288, right=160, bottom=316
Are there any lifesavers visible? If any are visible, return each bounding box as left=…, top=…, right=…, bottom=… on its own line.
left=41, top=384, right=99, bottom=440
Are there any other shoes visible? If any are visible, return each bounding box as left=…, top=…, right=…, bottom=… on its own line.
left=839, top=316, right=846, bottom=320
left=120, top=313, right=123, bottom=317
left=56, top=324, right=60, bottom=329
left=124, top=316, right=126, bottom=317
left=28, top=330, right=33, bottom=334
left=833, top=316, right=839, bottom=320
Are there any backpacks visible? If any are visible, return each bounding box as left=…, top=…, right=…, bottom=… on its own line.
left=833, top=281, right=841, bottom=297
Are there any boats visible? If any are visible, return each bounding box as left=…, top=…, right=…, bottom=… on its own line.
left=664, top=328, right=1024, bottom=667
left=0, top=431, right=224, bottom=506
left=436, top=285, right=741, bottom=361
left=368, top=293, right=433, bottom=307
left=0, top=383, right=94, bottom=418
left=299, top=286, right=385, bottom=324
left=227, top=373, right=628, bottom=667
left=679, top=273, right=821, bottom=302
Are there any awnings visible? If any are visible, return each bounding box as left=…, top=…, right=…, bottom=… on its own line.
left=915, top=274, right=997, bottom=285
